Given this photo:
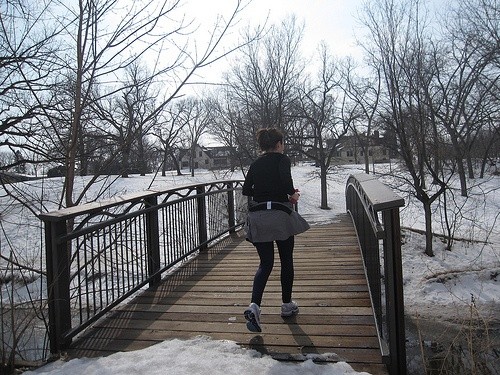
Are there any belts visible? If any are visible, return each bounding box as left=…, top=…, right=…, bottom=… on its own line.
left=248, top=203, right=292, bottom=216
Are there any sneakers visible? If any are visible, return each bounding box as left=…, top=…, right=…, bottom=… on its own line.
left=280, top=301, right=299, bottom=317
left=242, top=303, right=262, bottom=332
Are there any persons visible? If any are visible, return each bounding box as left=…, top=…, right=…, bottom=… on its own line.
left=243, top=126, right=311, bottom=333
